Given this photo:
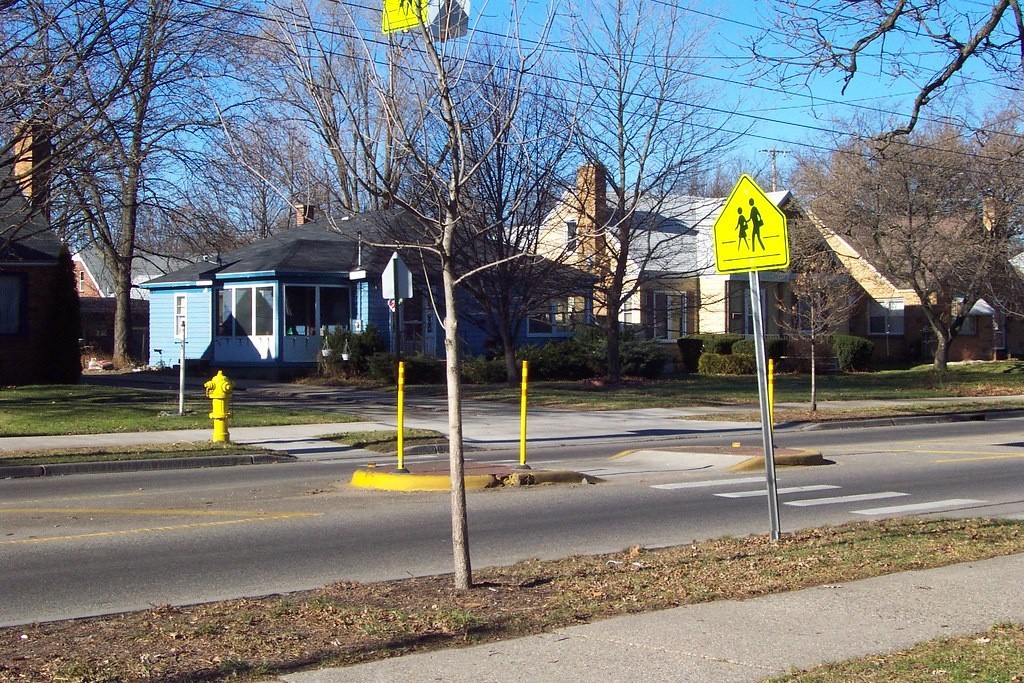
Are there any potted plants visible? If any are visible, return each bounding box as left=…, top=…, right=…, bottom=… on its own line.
left=321, top=335, right=333, bottom=357
left=341, top=339, right=352, bottom=361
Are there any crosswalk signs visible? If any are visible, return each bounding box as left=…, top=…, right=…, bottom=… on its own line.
left=382, top=0, right=428, bottom=35
left=712, top=174, right=788, bottom=275
